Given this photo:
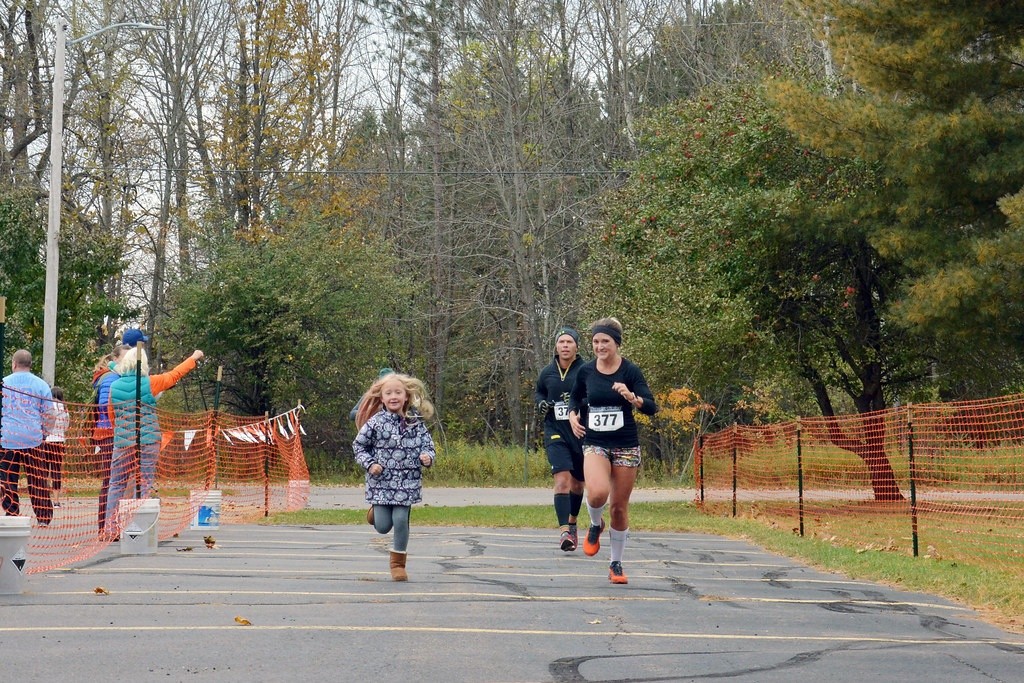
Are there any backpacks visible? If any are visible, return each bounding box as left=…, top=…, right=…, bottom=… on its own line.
left=79, top=370, right=112, bottom=437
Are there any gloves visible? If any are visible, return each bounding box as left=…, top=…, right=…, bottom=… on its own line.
left=538, top=399, right=550, bottom=415
left=559, top=391, right=571, bottom=404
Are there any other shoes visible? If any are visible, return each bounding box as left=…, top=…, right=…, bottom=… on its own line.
left=53, top=501, right=62, bottom=508
left=37, top=521, right=49, bottom=526
left=104, top=533, right=121, bottom=542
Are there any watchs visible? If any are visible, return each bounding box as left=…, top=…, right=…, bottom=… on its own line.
left=628, top=392, right=639, bottom=403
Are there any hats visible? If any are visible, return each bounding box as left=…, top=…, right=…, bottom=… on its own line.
left=379, top=368, right=393, bottom=377
left=555, top=327, right=579, bottom=344
left=122, top=328, right=148, bottom=346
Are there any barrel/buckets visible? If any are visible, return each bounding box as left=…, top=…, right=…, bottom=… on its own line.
left=190, top=490, right=223, bottom=530
left=289, top=482, right=309, bottom=509
left=116, top=499, right=159, bottom=554
left=0, top=515, right=31, bottom=594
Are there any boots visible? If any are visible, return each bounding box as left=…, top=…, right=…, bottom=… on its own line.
left=366, top=507, right=374, bottom=525
left=388, top=548, right=408, bottom=581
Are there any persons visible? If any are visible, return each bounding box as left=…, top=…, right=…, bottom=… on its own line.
left=351, top=368, right=437, bottom=581
left=534, top=325, right=593, bottom=551
left=0, top=349, right=54, bottom=527
left=43, top=387, right=71, bottom=509
left=91, top=329, right=204, bottom=542
left=569, top=317, right=656, bottom=585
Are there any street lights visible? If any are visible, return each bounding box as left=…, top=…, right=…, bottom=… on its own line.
left=41, top=16, right=167, bottom=390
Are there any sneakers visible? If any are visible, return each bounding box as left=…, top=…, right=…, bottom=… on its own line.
left=569, top=524, right=579, bottom=551
left=582, top=518, right=605, bottom=556
left=560, top=527, right=574, bottom=552
left=607, top=561, right=628, bottom=584
